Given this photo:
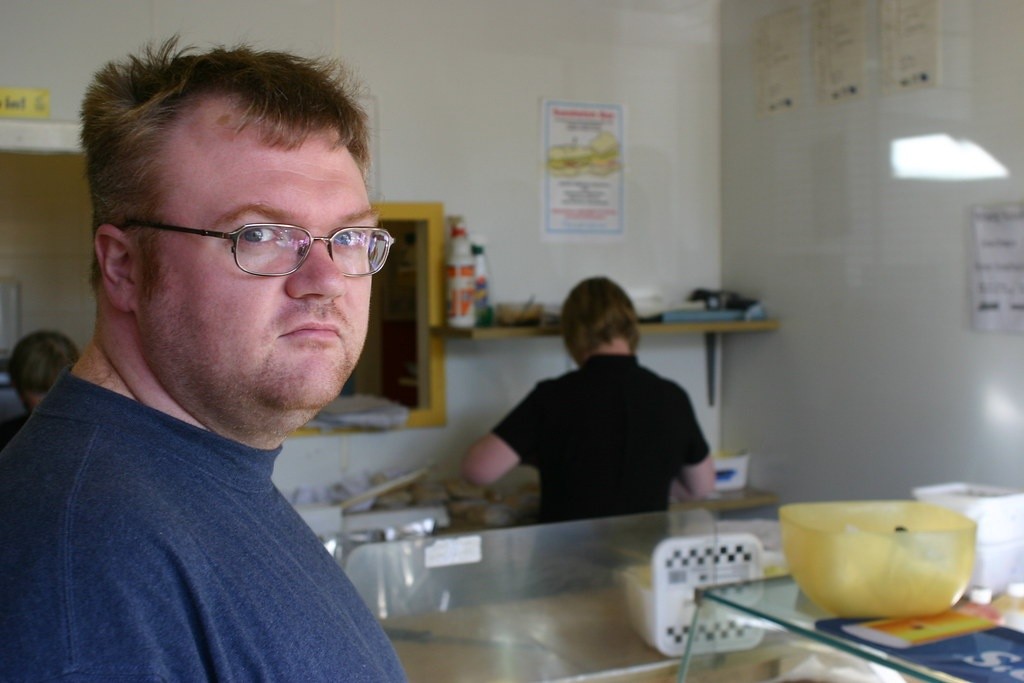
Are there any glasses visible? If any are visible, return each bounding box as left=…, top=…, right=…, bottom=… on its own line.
left=119, top=220, right=397, bottom=277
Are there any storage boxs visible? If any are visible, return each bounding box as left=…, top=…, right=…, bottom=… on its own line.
left=621, top=534, right=766, bottom=654
left=912, top=482, right=1024, bottom=545
left=962, top=541, right=1023, bottom=595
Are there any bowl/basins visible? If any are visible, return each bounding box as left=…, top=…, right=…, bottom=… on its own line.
left=498, top=303, right=543, bottom=327
left=780, top=500, right=978, bottom=617
left=714, top=455, right=749, bottom=490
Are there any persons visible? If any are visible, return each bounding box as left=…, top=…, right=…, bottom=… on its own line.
left=462, top=276, right=716, bottom=601
left=0, top=329, right=81, bottom=453
left=0, top=32, right=410, bottom=683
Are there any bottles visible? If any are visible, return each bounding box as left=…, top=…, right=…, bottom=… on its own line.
left=471, top=244, right=494, bottom=328
left=446, top=226, right=477, bottom=329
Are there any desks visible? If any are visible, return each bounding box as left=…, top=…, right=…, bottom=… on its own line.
left=377, top=585, right=780, bottom=683
